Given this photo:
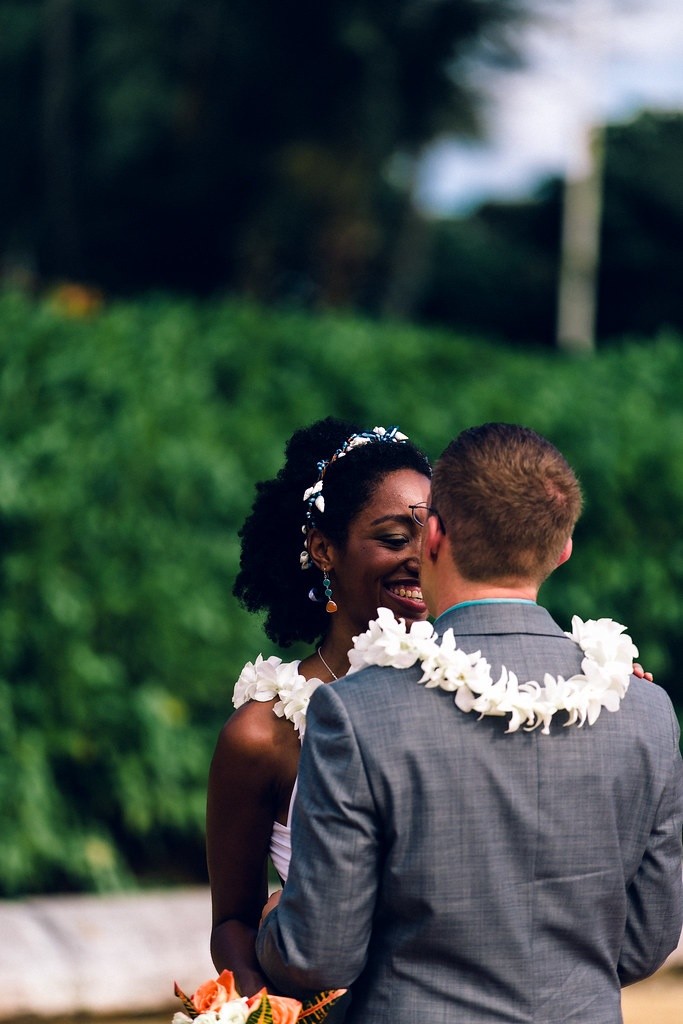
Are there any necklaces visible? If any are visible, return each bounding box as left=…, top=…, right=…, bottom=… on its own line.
left=344, top=607, right=640, bottom=736
left=231, top=653, right=324, bottom=747
left=318, top=646, right=340, bottom=681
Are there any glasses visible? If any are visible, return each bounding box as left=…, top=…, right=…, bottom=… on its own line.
left=409, top=502, right=446, bottom=534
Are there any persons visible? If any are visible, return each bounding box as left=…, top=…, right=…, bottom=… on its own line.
left=206, top=414, right=653, bottom=1023
left=255, top=420, right=683, bottom=1023
